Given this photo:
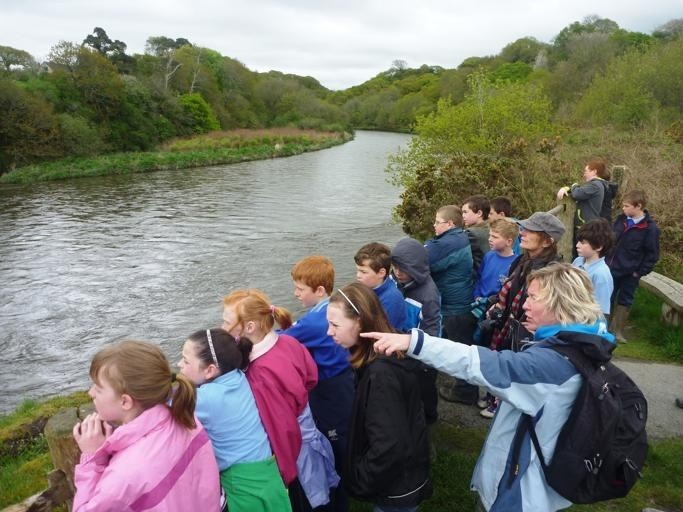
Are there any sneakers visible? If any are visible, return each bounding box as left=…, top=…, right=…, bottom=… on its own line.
left=480, top=403, right=496, bottom=418
left=478, top=397, right=491, bottom=408
left=440, top=387, right=472, bottom=404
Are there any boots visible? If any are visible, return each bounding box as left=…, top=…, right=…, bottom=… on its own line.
left=608, top=306, right=631, bottom=344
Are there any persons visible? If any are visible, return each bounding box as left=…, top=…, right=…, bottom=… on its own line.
left=170, top=327, right=292, bottom=511
left=72, top=340, right=223, bottom=511
left=274, top=255, right=358, bottom=451
left=460, top=159, right=661, bottom=418
left=354, top=242, right=424, bottom=341
left=389, top=235, right=444, bottom=423
left=220, top=289, right=342, bottom=511
left=424, top=205, right=479, bottom=404
left=326, top=281, right=433, bottom=512
left=358, top=262, right=648, bottom=512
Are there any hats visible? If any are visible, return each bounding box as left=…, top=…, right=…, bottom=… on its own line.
left=516, top=211, right=566, bottom=242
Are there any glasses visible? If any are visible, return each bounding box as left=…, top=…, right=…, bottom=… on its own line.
left=435, top=220, right=449, bottom=224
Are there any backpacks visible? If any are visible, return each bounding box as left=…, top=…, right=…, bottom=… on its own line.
left=522, top=343, right=647, bottom=504
